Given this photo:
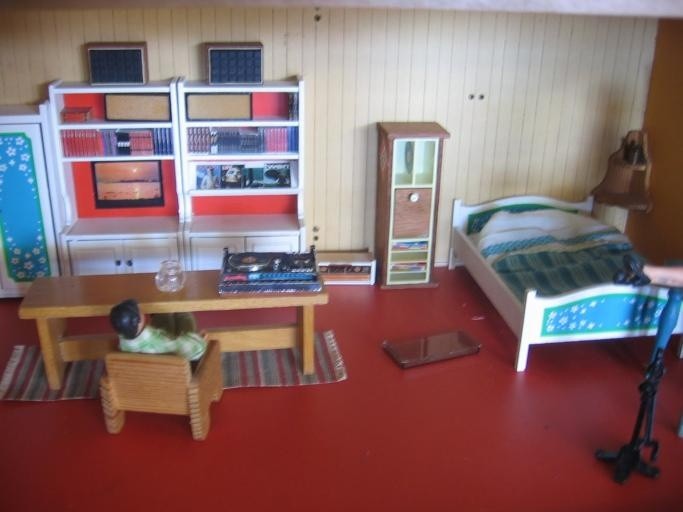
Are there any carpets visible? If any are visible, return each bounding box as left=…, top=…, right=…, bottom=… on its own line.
left=0, top=327, right=350, bottom=404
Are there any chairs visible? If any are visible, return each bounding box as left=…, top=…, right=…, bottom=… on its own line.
left=97, top=337, right=225, bottom=443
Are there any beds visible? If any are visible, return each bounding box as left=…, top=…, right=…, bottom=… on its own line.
left=449, top=190, right=683, bottom=375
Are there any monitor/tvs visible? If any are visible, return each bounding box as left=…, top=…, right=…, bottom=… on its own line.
left=91, top=160, right=164, bottom=208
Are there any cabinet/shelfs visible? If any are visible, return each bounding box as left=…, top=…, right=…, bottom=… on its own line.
left=178, top=78, right=307, bottom=272
left=590, top=127, right=655, bottom=216
left=47, top=75, right=183, bottom=274
left=376, top=121, right=454, bottom=291
left=314, top=251, right=376, bottom=287
left=1, top=105, right=61, bottom=302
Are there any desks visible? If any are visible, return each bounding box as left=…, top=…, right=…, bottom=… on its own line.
left=16, top=269, right=331, bottom=392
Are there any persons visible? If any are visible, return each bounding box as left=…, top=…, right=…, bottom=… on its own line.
left=108, top=299, right=208, bottom=361
left=200, top=168, right=216, bottom=189
left=220, top=166, right=240, bottom=182
left=264, top=170, right=287, bottom=184
